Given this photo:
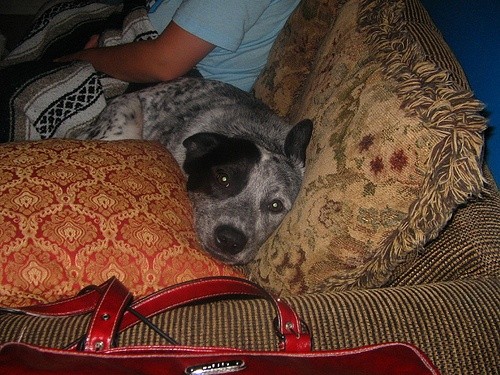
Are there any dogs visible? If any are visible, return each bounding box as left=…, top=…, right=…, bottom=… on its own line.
left=85, top=73, right=318, bottom=268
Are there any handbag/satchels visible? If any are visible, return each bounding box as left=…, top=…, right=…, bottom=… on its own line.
left=0, top=275, right=440, bottom=375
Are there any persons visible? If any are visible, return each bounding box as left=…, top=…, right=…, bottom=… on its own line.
left=0, top=1, right=302, bottom=131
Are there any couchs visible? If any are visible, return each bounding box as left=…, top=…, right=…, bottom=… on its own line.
left=0, top=0, right=500, bottom=375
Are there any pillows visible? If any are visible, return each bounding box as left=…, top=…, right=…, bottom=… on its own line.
left=0, top=0, right=487, bottom=307
